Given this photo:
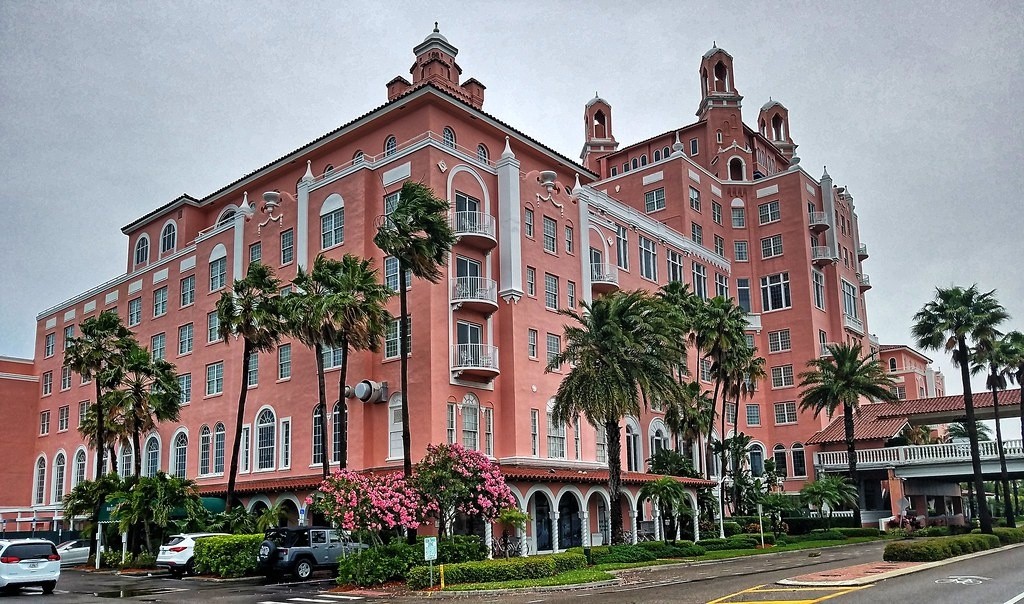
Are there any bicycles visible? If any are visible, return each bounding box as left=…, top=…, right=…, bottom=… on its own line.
left=491, top=529, right=529, bottom=557
left=611, top=527, right=656, bottom=545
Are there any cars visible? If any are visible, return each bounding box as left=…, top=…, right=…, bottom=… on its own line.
left=55, top=539, right=113, bottom=565
left=0, top=537, right=62, bottom=595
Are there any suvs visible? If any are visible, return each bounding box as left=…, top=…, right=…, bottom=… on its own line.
left=255, top=525, right=369, bottom=582
left=154, top=532, right=234, bottom=577
